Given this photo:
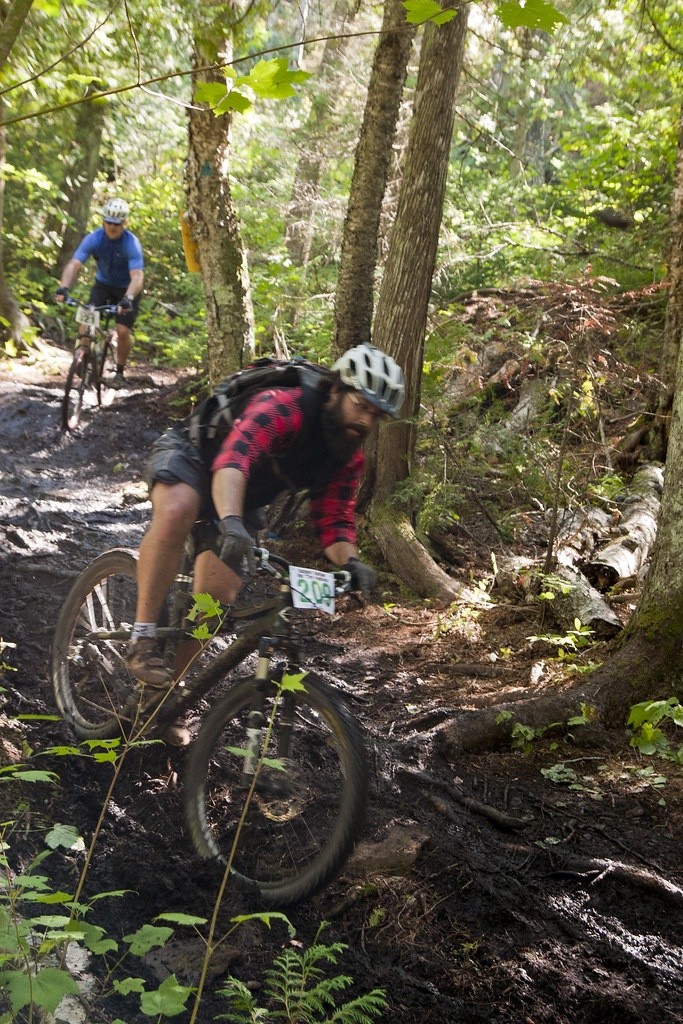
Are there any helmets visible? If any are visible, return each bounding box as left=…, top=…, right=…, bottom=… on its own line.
left=329, top=343, right=407, bottom=417
left=103, top=197, right=129, bottom=224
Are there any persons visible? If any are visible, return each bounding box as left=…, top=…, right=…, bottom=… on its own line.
left=55, top=197, right=145, bottom=387
left=118, top=342, right=407, bottom=691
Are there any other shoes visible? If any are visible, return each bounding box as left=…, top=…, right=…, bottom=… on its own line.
left=112, top=371, right=125, bottom=387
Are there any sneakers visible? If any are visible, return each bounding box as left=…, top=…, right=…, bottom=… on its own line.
left=164, top=711, right=191, bottom=745
left=125, top=637, right=171, bottom=690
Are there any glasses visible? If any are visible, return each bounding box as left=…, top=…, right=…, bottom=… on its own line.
left=345, top=393, right=385, bottom=425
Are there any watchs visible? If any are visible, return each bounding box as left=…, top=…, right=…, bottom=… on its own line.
left=124, top=293, right=135, bottom=302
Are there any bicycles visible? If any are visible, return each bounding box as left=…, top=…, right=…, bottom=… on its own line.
left=51, top=535, right=370, bottom=909
left=62, top=297, right=134, bottom=438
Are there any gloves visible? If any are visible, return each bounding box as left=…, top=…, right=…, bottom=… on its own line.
left=343, top=555, right=379, bottom=603
left=218, top=515, right=258, bottom=576
